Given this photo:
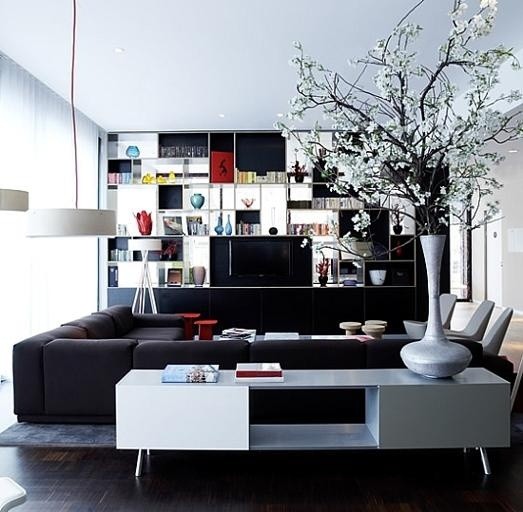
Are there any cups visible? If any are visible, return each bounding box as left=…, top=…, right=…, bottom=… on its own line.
left=191, top=266, right=205, bottom=285
left=160, top=146, right=208, bottom=157
left=369, top=270, right=387, bottom=286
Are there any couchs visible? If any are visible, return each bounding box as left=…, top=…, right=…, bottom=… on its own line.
left=11, top=304, right=517, bottom=426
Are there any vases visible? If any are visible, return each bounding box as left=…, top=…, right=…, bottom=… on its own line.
left=212, top=217, right=224, bottom=235
left=317, top=273, right=328, bottom=287
left=400, top=232, right=473, bottom=380
left=132, top=208, right=154, bottom=236
left=294, top=173, right=303, bottom=182
left=392, top=223, right=403, bottom=234
left=224, top=211, right=232, bottom=236
left=369, top=268, right=388, bottom=285
left=192, top=265, right=206, bottom=287
left=124, top=145, right=141, bottom=158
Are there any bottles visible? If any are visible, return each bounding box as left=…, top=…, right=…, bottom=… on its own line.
left=124, top=146, right=140, bottom=158
left=213, top=216, right=232, bottom=236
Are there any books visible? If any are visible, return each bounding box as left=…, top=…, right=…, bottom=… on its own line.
left=234, top=377, right=284, bottom=383
left=234, top=362, right=283, bottom=377
left=159, top=362, right=220, bottom=384
left=263, top=331, right=299, bottom=342
left=218, top=327, right=257, bottom=343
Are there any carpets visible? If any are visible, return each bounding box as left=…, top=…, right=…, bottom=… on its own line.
left=0, top=424, right=117, bottom=448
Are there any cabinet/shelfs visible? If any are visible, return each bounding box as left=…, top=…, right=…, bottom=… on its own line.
left=112, top=367, right=514, bottom=478
left=107, top=288, right=211, bottom=326
left=211, top=288, right=313, bottom=332
left=104, top=129, right=419, bottom=287
left=313, top=289, right=417, bottom=333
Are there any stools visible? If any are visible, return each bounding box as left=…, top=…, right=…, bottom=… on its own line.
left=176, top=312, right=201, bottom=337
left=360, top=323, right=386, bottom=338
left=364, top=319, right=388, bottom=329
left=337, top=320, right=361, bottom=335
left=193, top=319, right=218, bottom=340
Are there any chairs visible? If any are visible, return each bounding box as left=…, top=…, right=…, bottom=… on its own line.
left=402, top=291, right=457, bottom=337
left=480, top=307, right=515, bottom=355
left=443, top=298, right=496, bottom=342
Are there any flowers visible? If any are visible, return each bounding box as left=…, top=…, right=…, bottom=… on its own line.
left=273, top=1, right=521, bottom=264
left=312, top=256, right=330, bottom=278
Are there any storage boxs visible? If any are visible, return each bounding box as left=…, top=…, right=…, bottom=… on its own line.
left=340, top=239, right=374, bottom=260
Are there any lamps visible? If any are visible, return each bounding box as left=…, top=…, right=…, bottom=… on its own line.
left=25, top=0, right=117, bottom=240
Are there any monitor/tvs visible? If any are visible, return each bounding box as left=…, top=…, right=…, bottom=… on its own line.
left=229, top=239, right=294, bottom=278
